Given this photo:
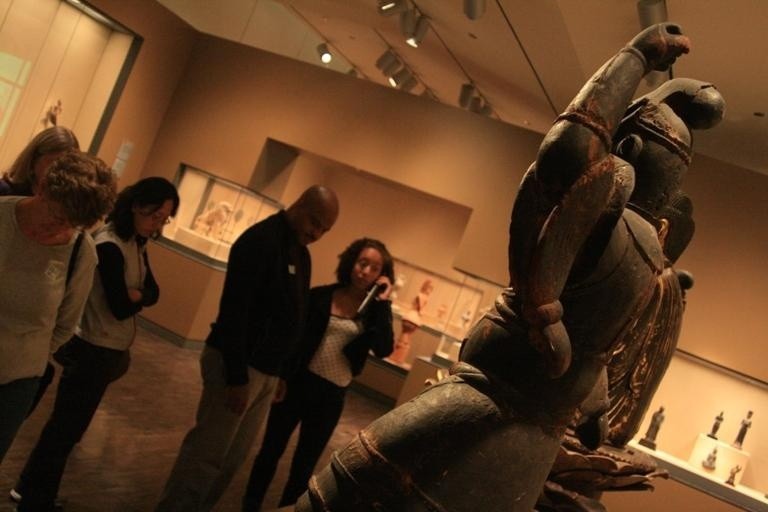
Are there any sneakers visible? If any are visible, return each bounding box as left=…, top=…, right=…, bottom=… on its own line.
left=9, top=479, right=68, bottom=508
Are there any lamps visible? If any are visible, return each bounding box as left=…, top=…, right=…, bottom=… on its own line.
left=316, top=2, right=493, bottom=119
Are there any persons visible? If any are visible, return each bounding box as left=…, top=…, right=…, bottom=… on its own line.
left=10, top=177, right=178, bottom=512
left=568, top=188, right=693, bottom=448
left=0, top=125, right=79, bottom=197
left=242, top=236, right=396, bottom=511
left=644, top=406, right=666, bottom=440
left=709, top=408, right=724, bottom=435
left=409, top=279, right=435, bottom=316
left=727, top=464, right=741, bottom=483
left=733, top=410, right=754, bottom=448
left=45, top=98, right=63, bottom=126
left=704, top=449, right=718, bottom=468
left=296, top=23, right=726, bottom=510
left=155, top=185, right=338, bottom=511
left=0, top=148, right=118, bottom=469
